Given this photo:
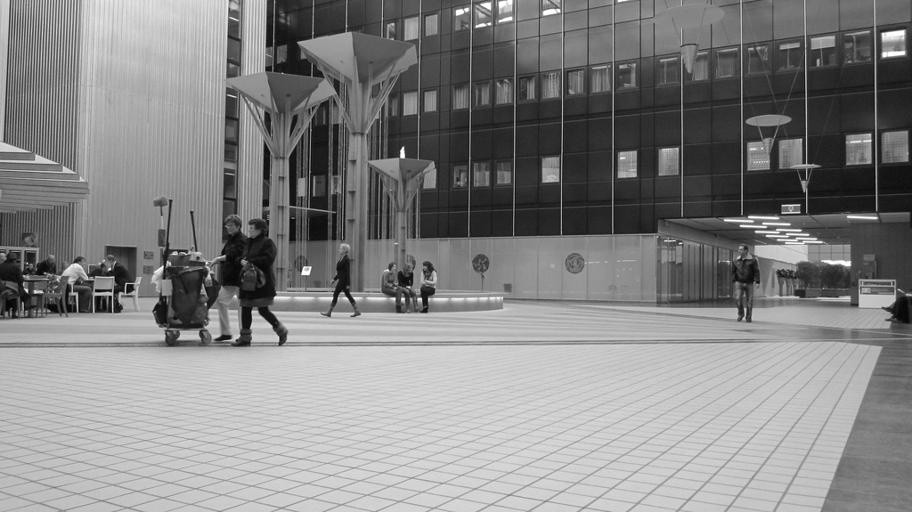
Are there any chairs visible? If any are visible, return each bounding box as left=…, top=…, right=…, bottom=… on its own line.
left=2, top=264, right=143, bottom=319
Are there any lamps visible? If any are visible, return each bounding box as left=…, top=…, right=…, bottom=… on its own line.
left=743, top=0, right=847, bottom=195
left=646, top=1, right=726, bottom=75
left=732, top=45, right=805, bottom=157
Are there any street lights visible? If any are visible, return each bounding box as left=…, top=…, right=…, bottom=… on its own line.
left=153, top=195, right=167, bottom=261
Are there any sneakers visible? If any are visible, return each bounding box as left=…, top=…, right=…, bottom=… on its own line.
left=277, top=325, right=288, bottom=347
left=350, top=311, right=361, bottom=317
left=214, top=333, right=232, bottom=342
left=320, top=312, right=331, bottom=317
left=231, top=339, right=251, bottom=347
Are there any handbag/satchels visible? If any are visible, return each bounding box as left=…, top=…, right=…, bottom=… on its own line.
left=239, top=263, right=258, bottom=292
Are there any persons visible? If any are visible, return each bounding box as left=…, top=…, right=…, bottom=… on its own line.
left=881, top=295, right=912, bottom=322
left=0, top=251, right=134, bottom=319
left=321, top=243, right=360, bottom=317
left=230, top=218, right=288, bottom=347
left=397, top=262, right=419, bottom=314
left=207, top=214, right=242, bottom=341
left=731, top=244, right=761, bottom=323
left=421, top=261, right=437, bottom=312
left=382, top=263, right=402, bottom=314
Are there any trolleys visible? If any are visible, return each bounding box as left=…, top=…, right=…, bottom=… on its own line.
left=151, top=254, right=220, bottom=348
